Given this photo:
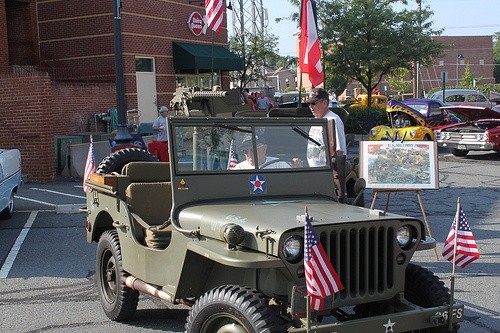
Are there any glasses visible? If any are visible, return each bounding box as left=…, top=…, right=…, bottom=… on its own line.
left=306, top=98, right=324, bottom=106
left=242, top=145, right=266, bottom=155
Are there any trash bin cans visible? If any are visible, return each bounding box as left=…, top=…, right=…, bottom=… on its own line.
left=80, top=132, right=110, bottom=143
left=53, top=135, right=81, bottom=174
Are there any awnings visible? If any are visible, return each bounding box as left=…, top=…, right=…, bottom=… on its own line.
left=172, top=42, right=245, bottom=73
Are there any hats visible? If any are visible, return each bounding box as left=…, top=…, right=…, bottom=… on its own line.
left=328, top=89, right=332, bottom=93
left=243, top=88, right=250, bottom=94
left=159, top=105, right=169, bottom=113
left=304, top=88, right=329, bottom=103
left=241, top=130, right=270, bottom=145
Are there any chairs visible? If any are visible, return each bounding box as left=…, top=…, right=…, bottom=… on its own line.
left=121, top=162, right=172, bottom=226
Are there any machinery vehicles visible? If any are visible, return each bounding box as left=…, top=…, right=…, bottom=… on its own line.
left=171, top=83, right=267, bottom=118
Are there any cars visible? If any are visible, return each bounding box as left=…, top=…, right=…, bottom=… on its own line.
left=434, top=102, right=500, bottom=155
left=369, top=98, right=462, bottom=141
left=430, top=88, right=497, bottom=109
left=272, top=91, right=308, bottom=107
left=349, top=95, right=388, bottom=109
left=0, top=148, right=23, bottom=219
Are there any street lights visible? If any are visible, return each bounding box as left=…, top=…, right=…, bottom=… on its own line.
left=457, top=49, right=464, bottom=84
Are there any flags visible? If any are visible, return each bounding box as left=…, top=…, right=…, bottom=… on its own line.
left=298, top=0, right=325, bottom=86
left=303, top=216, right=344, bottom=313
left=229, top=138, right=238, bottom=169
left=82, top=138, right=96, bottom=192
left=205, top=0, right=224, bottom=32
left=441, top=205, right=480, bottom=269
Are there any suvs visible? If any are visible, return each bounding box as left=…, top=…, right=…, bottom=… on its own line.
left=80, top=118, right=465, bottom=333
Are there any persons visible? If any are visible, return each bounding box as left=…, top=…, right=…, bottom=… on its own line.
left=306, top=87, right=347, bottom=167
left=244, top=88, right=272, bottom=110
left=153, top=105, right=170, bottom=141
left=231, top=129, right=303, bottom=168
left=304, top=86, right=337, bottom=100
